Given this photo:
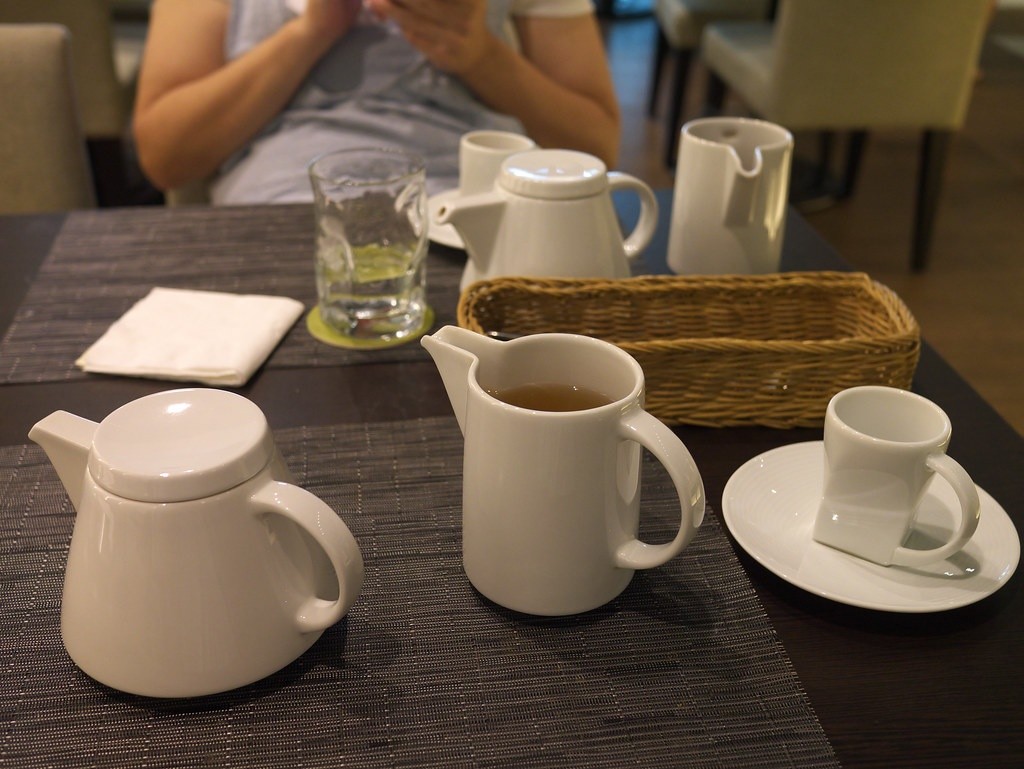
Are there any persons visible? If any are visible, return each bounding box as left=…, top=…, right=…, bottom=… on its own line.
left=129, top=0, right=623, bottom=205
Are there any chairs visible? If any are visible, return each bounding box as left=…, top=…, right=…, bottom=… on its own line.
left=648, top=0, right=998, bottom=272
left=0, top=0, right=133, bottom=216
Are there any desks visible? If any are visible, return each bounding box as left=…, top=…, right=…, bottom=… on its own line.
left=0, top=183, right=1024, bottom=769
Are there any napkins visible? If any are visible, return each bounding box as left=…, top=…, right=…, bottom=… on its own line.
left=74, top=285, right=304, bottom=386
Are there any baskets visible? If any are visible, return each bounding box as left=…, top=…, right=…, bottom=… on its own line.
left=456, top=270, right=923, bottom=431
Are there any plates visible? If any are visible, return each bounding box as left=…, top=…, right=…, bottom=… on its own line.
left=722, top=442, right=1021, bottom=612
left=409, top=189, right=467, bottom=245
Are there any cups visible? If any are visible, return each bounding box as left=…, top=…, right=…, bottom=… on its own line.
left=306, top=148, right=427, bottom=335
left=816, top=386, right=979, bottom=568
left=460, top=131, right=534, bottom=192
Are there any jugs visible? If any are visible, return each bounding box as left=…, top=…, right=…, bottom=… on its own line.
left=419, top=324, right=704, bottom=615
left=668, top=119, right=793, bottom=274
left=30, top=388, right=363, bottom=698
left=432, top=149, right=659, bottom=293
